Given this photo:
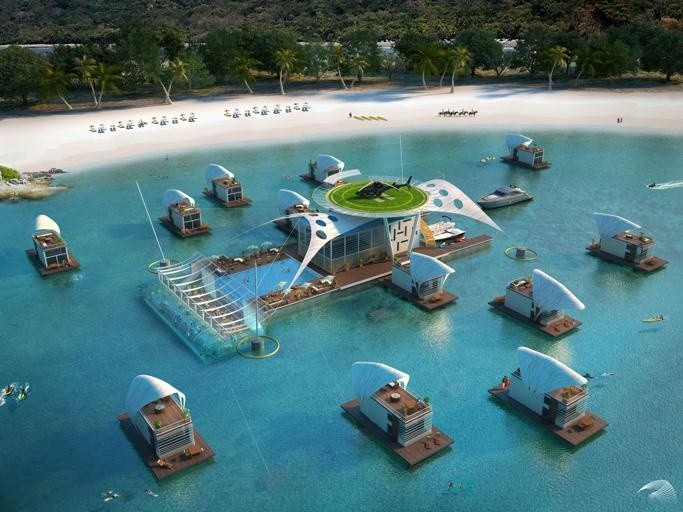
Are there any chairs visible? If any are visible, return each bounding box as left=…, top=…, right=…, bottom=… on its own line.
left=157, top=459, right=172, bottom=469
left=576, top=415, right=595, bottom=430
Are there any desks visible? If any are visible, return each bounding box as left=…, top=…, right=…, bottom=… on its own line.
left=183, top=446, right=202, bottom=458
